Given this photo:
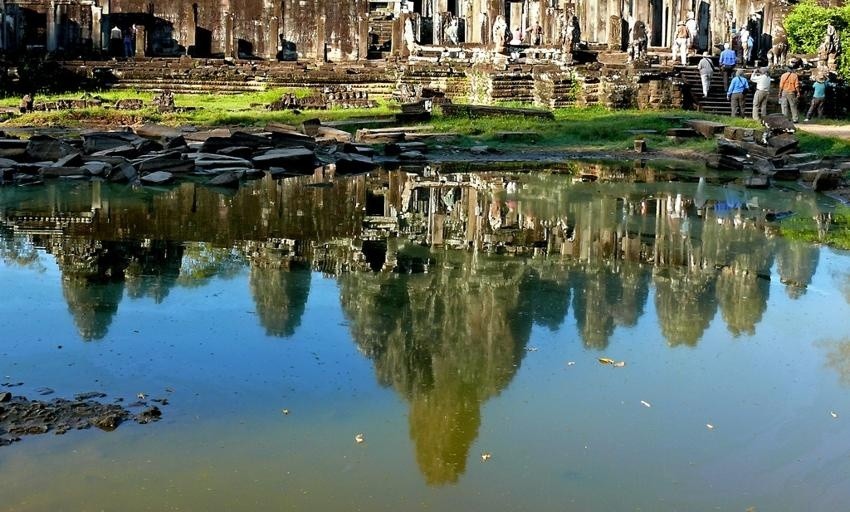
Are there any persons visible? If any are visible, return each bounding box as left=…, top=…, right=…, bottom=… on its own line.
left=719, top=42, right=737, bottom=93
left=671, top=22, right=691, bottom=66
left=778, top=66, right=801, bottom=122
left=804, top=74, right=827, bottom=122
left=751, top=67, right=771, bottom=121
left=123, top=25, right=134, bottom=59
left=739, top=25, right=751, bottom=69
left=686, top=21, right=700, bottom=54
left=527, top=21, right=543, bottom=47
left=727, top=68, right=749, bottom=119
left=620, top=21, right=631, bottom=52
left=514, top=25, right=522, bottom=45
left=109, top=23, right=123, bottom=60
left=698, top=51, right=713, bottom=97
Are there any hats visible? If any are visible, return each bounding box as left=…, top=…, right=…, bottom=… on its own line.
left=815, top=75, right=825, bottom=82
left=699, top=52, right=711, bottom=57
left=677, top=21, right=686, bottom=26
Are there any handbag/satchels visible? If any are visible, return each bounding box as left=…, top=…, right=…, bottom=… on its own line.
left=742, top=88, right=748, bottom=94
